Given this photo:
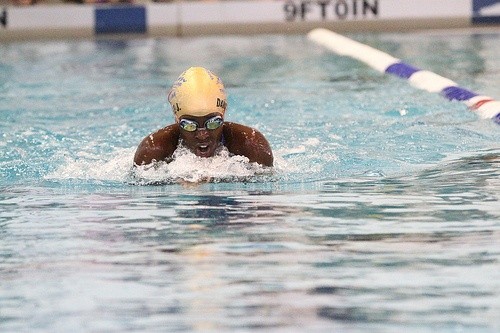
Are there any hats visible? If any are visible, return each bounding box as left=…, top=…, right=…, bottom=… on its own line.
left=168, top=67, right=227, bottom=118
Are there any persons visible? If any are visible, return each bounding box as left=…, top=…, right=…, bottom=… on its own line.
left=133, top=67, right=273, bottom=168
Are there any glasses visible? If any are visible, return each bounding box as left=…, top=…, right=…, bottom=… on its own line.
left=176, top=116, right=224, bottom=132
